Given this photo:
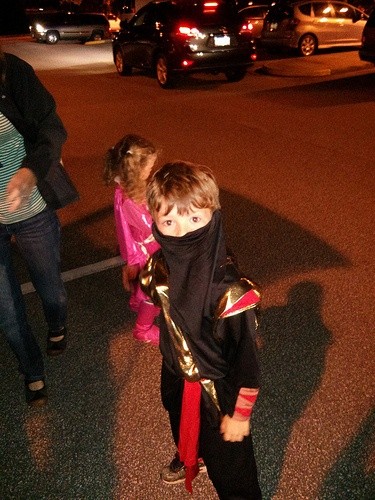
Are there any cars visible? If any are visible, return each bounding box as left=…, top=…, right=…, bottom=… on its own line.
left=99, top=13, right=122, bottom=33
left=260, top=0, right=370, bottom=57
left=237, top=5, right=273, bottom=38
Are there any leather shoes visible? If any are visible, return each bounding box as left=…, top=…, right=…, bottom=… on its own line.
left=25, top=381, right=47, bottom=407
left=45, top=324, right=68, bottom=358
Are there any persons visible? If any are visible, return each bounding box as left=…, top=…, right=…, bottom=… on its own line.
left=0, top=52, right=68, bottom=407
left=104, top=136, right=163, bottom=345
left=138, top=160, right=263, bottom=500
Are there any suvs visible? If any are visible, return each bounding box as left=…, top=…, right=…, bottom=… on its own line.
left=28, top=9, right=110, bottom=46
left=111, top=0, right=258, bottom=88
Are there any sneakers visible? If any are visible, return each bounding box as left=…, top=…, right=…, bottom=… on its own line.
left=160, top=452, right=208, bottom=484
